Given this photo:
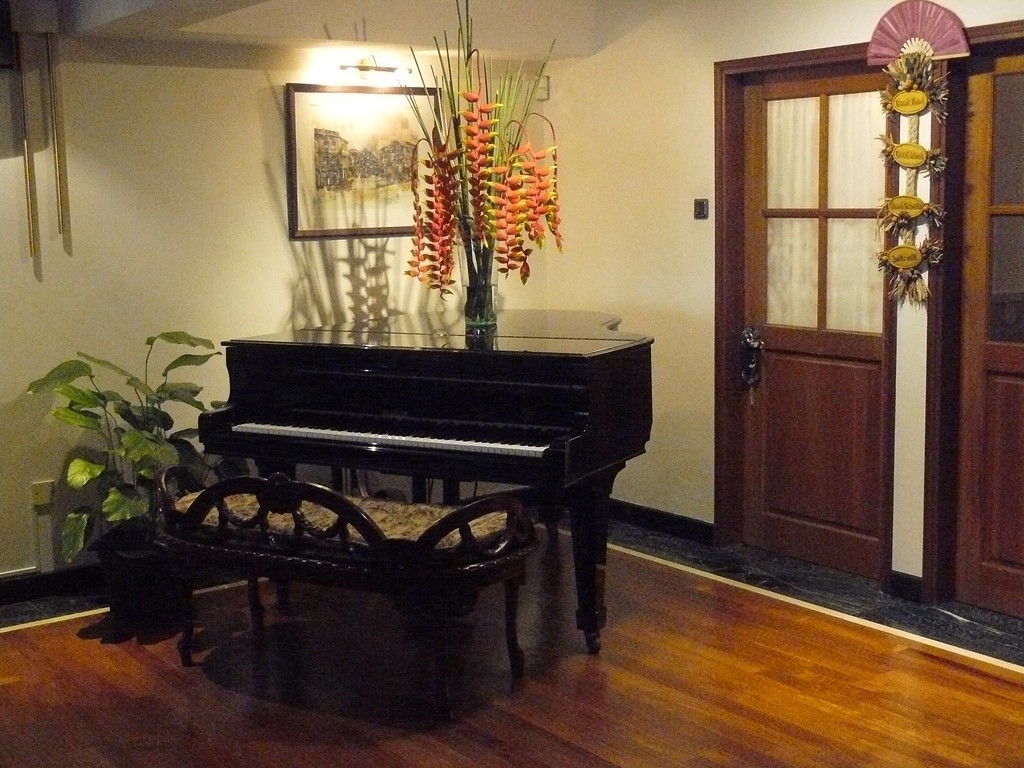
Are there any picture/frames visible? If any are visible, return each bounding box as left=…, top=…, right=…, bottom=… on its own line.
left=284, top=82, right=445, bottom=241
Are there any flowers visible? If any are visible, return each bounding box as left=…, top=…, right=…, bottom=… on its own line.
left=399, top=1, right=564, bottom=325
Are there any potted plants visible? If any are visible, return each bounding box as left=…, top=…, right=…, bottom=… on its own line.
left=19, top=331, right=224, bottom=631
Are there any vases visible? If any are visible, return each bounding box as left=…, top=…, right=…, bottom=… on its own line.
left=455, top=215, right=502, bottom=330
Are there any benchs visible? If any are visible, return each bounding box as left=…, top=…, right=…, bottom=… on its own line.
left=146, top=459, right=542, bottom=716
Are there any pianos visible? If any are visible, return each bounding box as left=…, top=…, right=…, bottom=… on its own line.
left=197, top=308, right=658, bottom=656
